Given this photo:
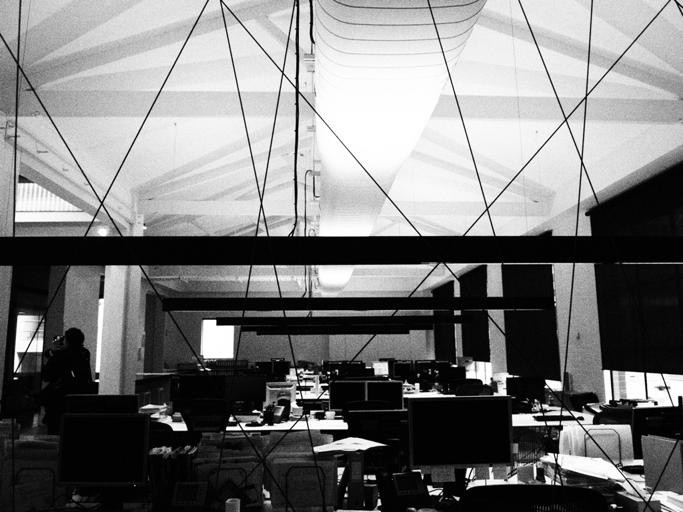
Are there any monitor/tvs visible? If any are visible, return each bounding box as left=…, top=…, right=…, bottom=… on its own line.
left=347, top=409, right=408, bottom=481
left=66, top=395, right=140, bottom=413
left=330, top=380, right=367, bottom=419
left=408, top=395, right=513, bottom=491
left=366, top=381, right=404, bottom=410
left=56, top=412, right=148, bottom=511
left=627, top=406, right=682, bottom=460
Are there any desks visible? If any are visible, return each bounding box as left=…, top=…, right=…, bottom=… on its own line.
left=403, top=393, right=456, bottom=399
left=295, top=391, right=330, bottom=402
left=512, top=411, right=594, bottom=454
left=157, top=414, right=349, bottom=435
left=296, top=382, right=329, bottom=391
left=303, top=375, right=316, bottom=381
left=304, top=371, right=314, bottom=374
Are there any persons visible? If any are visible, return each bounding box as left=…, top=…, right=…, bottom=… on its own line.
left=43, top=329, right=94, bottom=421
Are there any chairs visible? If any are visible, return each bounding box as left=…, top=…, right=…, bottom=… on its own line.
left=456, top=384, right=482, bottom=395
left=568, top=423, right=634, bottom=460
left=452, top=379, right=482, bottom=390
left=458, top=485, right=609, bottom=512
left=343, top=401, right=397, bottom=438
